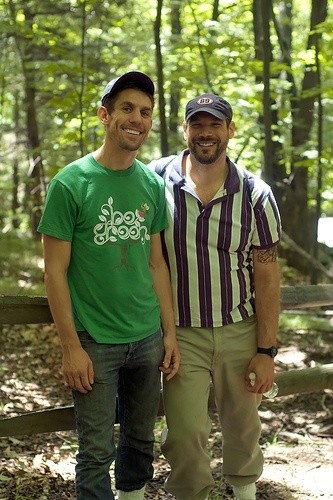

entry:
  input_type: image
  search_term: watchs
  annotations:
[256,346,278,358]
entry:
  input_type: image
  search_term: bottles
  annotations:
[249,373,279,399]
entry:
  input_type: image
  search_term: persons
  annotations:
[144,94,282,500]
[36,71,169,500]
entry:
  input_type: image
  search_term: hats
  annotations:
[100,71,156,102]
[183,94,234,122]
